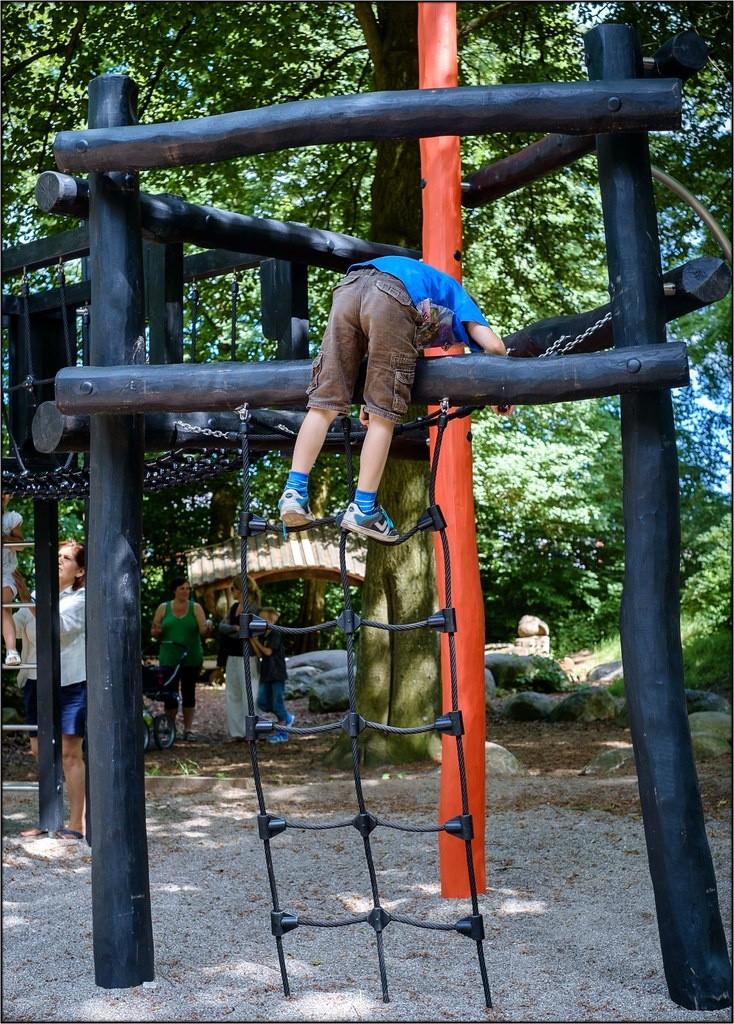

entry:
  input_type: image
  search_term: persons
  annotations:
[11,542,85,839]
[277,257,512,541]
[210,575,295,743]
[1,494,24,665]
[150,578,212,742]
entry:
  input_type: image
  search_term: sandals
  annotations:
[5,649,21,665]
[183,731,198,742]
[166,727,184,740]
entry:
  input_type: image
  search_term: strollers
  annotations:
[142,640,188,752]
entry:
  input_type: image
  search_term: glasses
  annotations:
[231,587,238,592]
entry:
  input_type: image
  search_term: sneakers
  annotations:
[278,489,314,532]
[266,732,289,744]
[341,503,399,541]
[286,713,294,727]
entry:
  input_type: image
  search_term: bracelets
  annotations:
[30,597,35,603]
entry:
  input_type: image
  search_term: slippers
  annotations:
[20,828,83,839]
